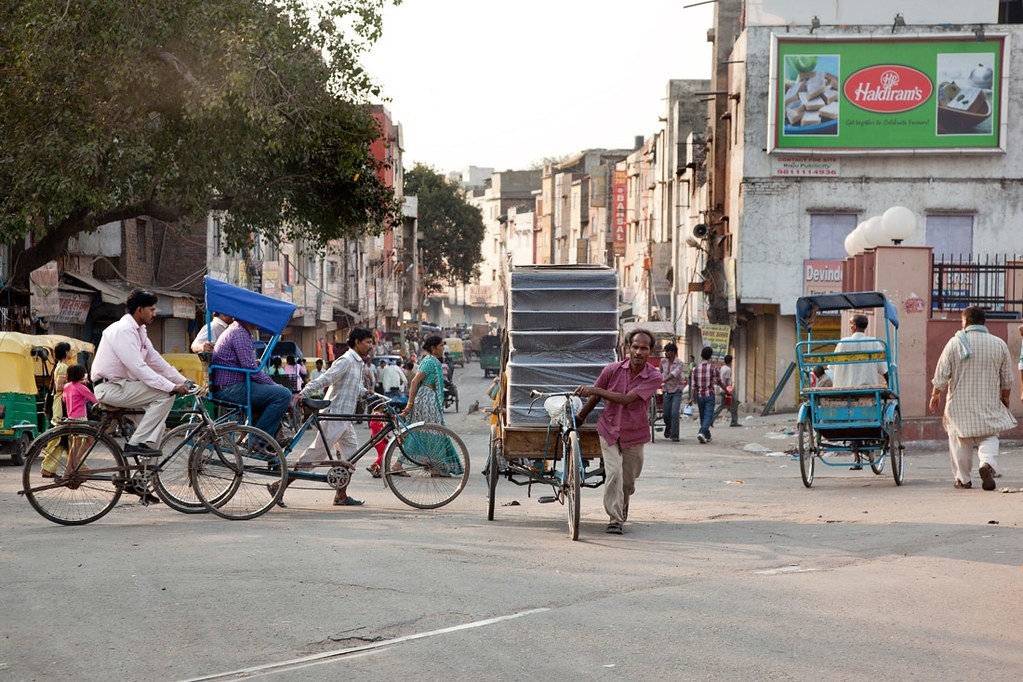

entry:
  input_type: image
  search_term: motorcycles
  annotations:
[160,353,216,424]
[0,330,98,467]
[441,337,465,368]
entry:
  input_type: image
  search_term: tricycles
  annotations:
[255,340,326,433]
[481,265,623,543]
[615,321,681,444]
[442,378,458,413]
[796,292,907,488]
[480,335,502,378]
[153,275,472,510]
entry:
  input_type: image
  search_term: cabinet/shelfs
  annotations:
[507,269,618,426]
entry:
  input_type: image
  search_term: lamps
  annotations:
[809,15,821,33]
[891,13,907,33]
[845,206,915,257]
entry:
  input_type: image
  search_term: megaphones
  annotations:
[693,223,708,238]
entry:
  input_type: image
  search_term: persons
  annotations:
[813,365,831,386]
[930,305,1014,491]
[572,331,663,534]
[40,288,505,506]
[1018,339,1023,403]
[660,343,742,445]
[832,316,889,470]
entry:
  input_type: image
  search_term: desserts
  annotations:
[938,81,986,113]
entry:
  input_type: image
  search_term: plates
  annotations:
[782,117,837,133]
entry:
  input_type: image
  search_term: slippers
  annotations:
[333,496,366,505]
[390,471,411,478]
[266,482,288,509]
[431,472,452,478]
[42,473,62,478]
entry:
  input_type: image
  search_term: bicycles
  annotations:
[22,382,289,526]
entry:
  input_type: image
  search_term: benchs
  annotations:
[797,338,892,425]
[185,352,282,464]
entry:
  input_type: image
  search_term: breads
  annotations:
[784,68,839,126]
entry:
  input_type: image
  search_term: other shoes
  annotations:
[979,463,996,490]
[664,430,670,438]
[366,464,379,478]
[954,480,972,489]
[243,438,277,458]
[606,523,624,535]
[623,504,629,523]
[122,443,163,458]
[705,433,712,442]
[697,431,708,444]
[672,437,680,442]
[124,479,160,503]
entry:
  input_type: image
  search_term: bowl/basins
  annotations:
[937,97,991,131]
